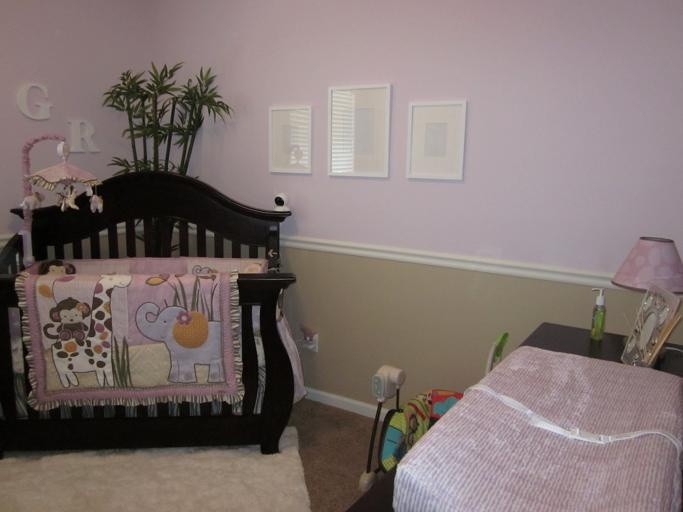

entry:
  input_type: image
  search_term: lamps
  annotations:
[611,236,683,294]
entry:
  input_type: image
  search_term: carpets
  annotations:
[1,427,312,512]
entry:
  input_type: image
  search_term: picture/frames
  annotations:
[267,84,466,181]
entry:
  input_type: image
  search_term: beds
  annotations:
[1,172,295,457]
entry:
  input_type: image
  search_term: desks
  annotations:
[343,322,682,512]
